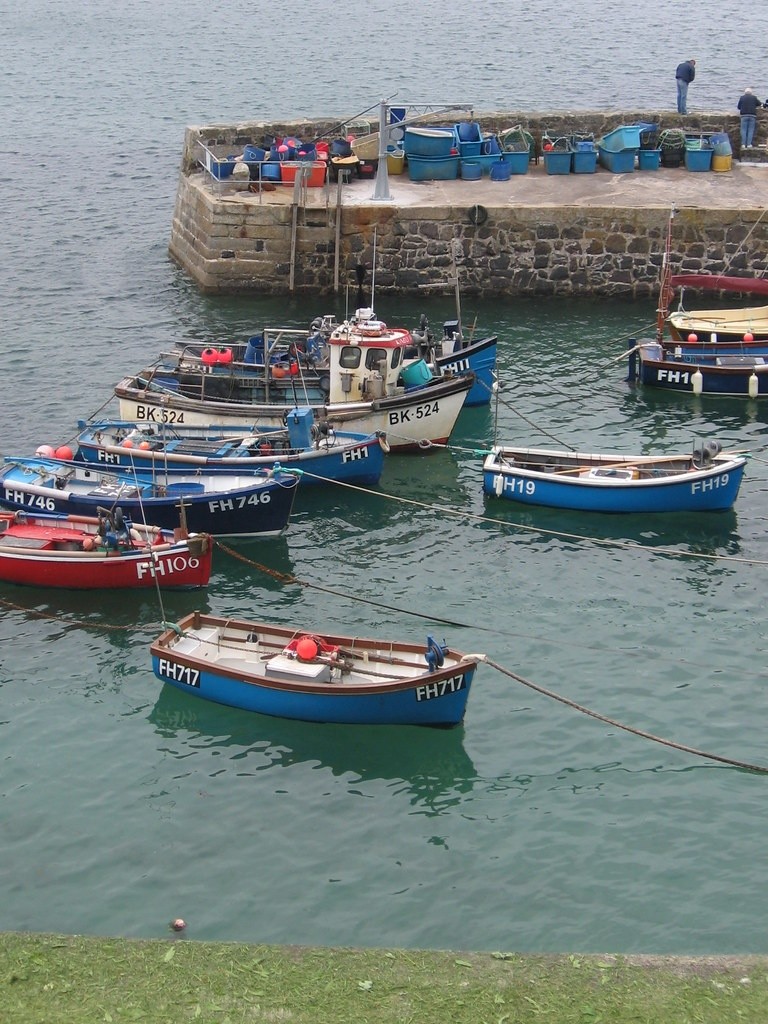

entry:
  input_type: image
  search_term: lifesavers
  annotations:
[356,320,387,332]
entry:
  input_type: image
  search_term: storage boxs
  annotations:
[211,120,734,193]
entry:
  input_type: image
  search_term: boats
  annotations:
[482,434,748,513]
[159,225,498,406]
[1,492,214,589]
[117,317,475,450]
[149,610,479,726]
[76,416,391,488]
[654,201,768,337]
[0,448,300,538]
[637,336,768,400]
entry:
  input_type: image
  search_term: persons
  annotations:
[419,313,429,331]
[737,88,763,149]
[675,59,696,115]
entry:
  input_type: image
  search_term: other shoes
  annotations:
[681,111,692,115]
[741,144,746,149]
[747,144,753,148]
